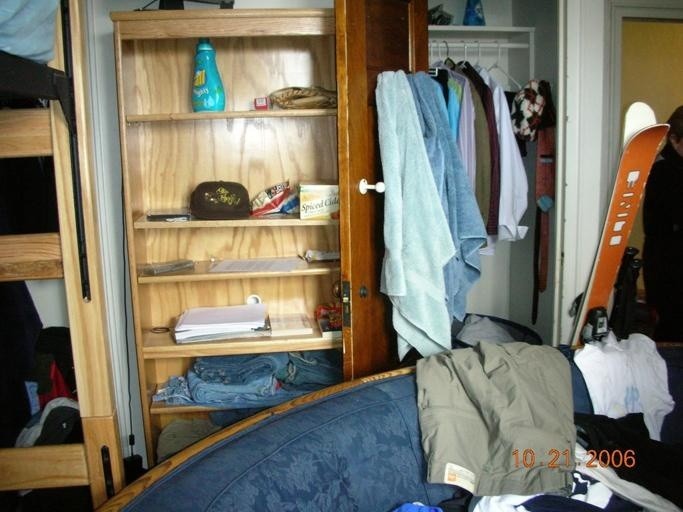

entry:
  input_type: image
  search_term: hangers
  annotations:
[423,39,524,96]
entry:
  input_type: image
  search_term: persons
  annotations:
[642,103,683,348]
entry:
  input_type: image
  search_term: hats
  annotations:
[511,80,545,143]
[189,181,249,220]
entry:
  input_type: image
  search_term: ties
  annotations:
[535,127,555,292]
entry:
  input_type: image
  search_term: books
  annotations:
[172,305,343,345]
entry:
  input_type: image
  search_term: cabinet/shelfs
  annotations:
[107,8,350,468]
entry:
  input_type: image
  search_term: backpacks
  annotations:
[14,397,84,497]
[33,327,78,411]
